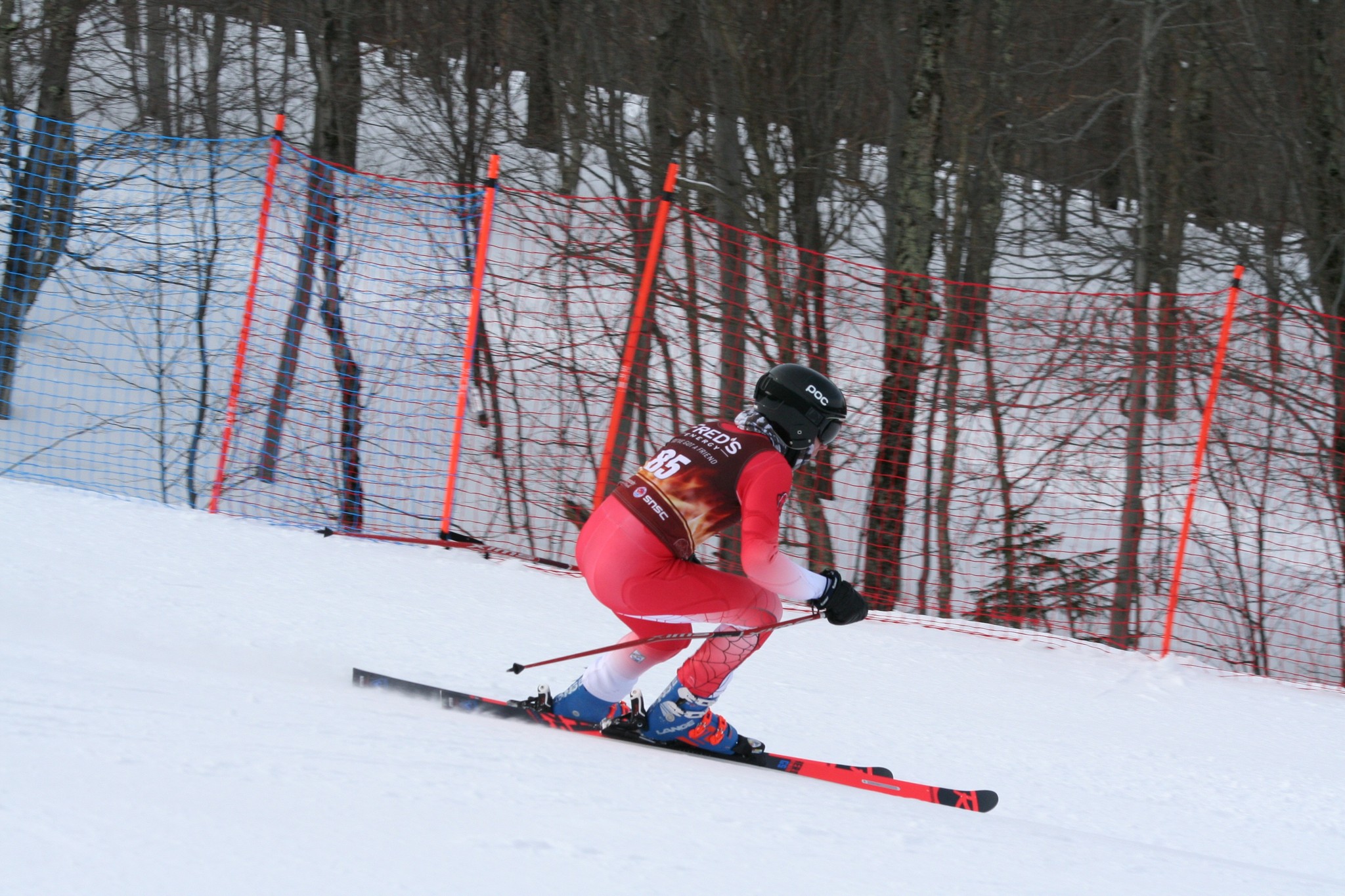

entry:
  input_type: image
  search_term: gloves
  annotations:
[807,568,869,625]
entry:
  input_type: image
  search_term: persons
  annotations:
[551,363,869,754]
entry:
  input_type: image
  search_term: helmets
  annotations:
[754,363,847,450]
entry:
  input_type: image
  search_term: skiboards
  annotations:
[350,665,1000,815]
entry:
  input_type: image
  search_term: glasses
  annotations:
[818,417,844,446]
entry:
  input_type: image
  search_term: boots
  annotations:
[639,674,738,755]
[549,674,633,724]
